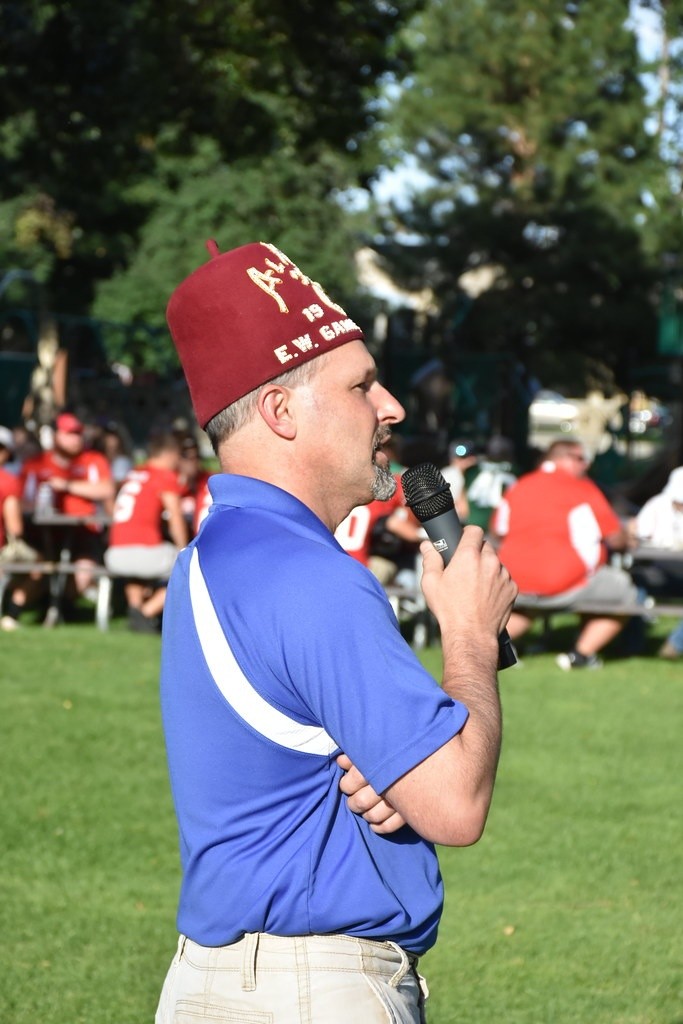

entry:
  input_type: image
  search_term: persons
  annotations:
[153,236,517,1023]
[1,414,683,672]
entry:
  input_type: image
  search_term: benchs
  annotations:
[382,588,683,663]
[0,561,169,632]
[478,535,681,566]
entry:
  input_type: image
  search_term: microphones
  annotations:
[399,462,517,669]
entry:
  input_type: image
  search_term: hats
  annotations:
[165,238,366,429]
